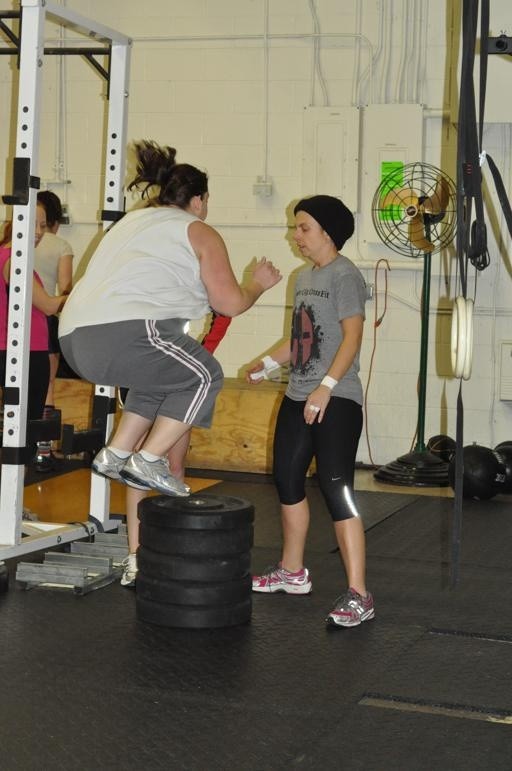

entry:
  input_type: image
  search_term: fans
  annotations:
[373,162,464,490]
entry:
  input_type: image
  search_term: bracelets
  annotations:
[321,376,338,391]
[260,355,273,369]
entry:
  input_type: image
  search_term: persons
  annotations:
[0,199,71,449]
[34,191,74,460]
[245,195,376,628]
[57,137,283,497]
[120,194,232,586]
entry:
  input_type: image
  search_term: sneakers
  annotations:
[120,553,138,586]
[36,455,55,472]
[92,448,190,497]
[251,561,313,594]
[325,588,375,628]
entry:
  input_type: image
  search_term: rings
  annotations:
[314,407,320,413]
[309,405,314,410]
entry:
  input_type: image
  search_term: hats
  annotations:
[295,195,354,251]
